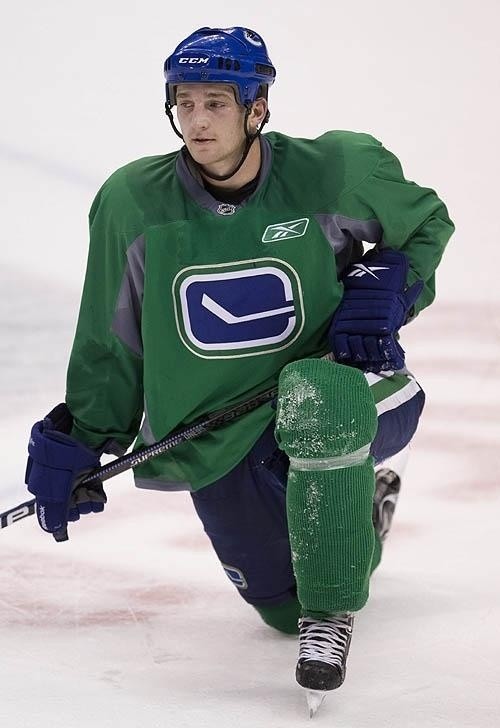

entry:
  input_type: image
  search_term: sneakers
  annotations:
[369,465,403,545]
[292,601,354,694]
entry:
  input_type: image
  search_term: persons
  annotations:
[24,20,459,693]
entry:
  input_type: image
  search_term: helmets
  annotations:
[160,22,276,110]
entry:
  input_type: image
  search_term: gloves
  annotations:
[22,398,112,543]
[327,246,424,376]
